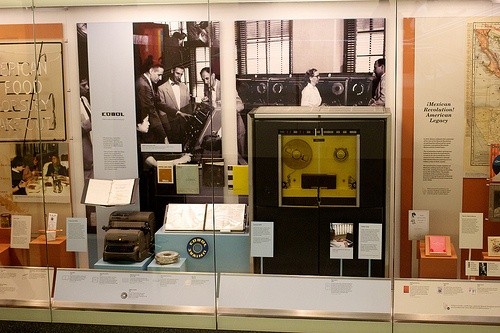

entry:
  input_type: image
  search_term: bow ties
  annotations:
[209,88,215,92]
[171,82,179,86]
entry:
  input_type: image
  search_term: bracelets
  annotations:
[18,184,20,189]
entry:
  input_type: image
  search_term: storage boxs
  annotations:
[94,251,155,269]
[146,224,252,271]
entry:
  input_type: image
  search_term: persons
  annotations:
[135,61,246,230]
[300,68,329,106]
[330,229,348,248]
[79,73,97,233]
[11,154,41,195]
[47,154,68,176]
[368,59,386,106]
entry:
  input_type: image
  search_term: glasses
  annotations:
[313,75,319,78]
